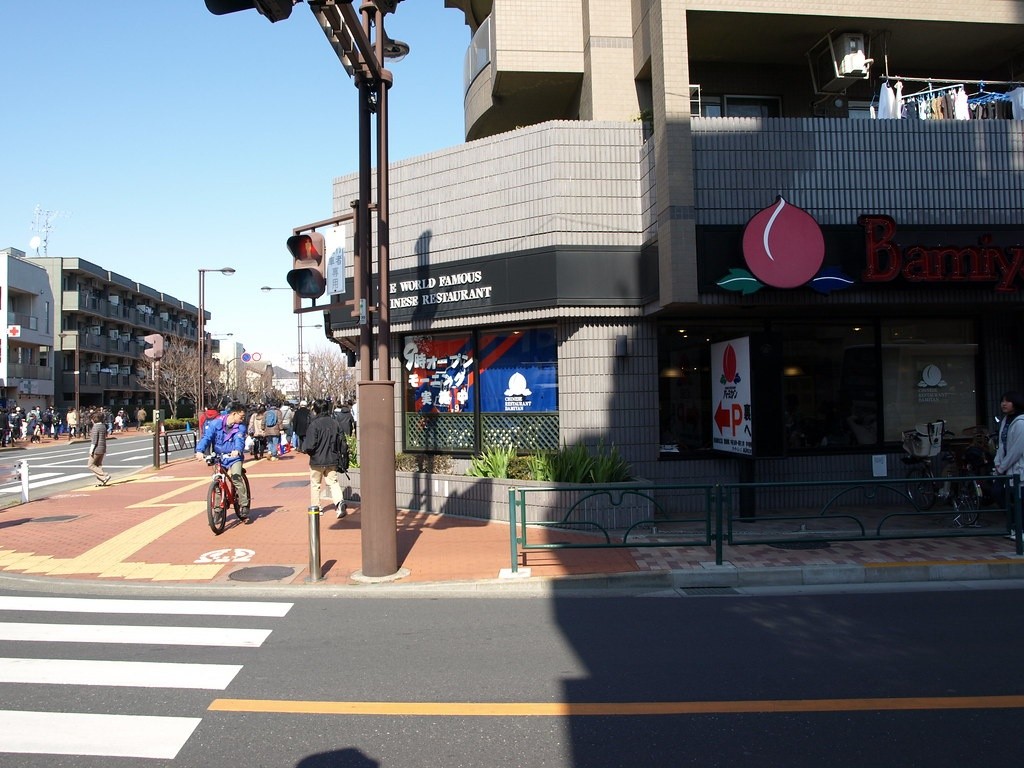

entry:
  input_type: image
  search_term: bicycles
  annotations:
[902,428,987,528]
[199,453,254,535]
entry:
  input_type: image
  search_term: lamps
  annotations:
[783,353,805,377]
[660,342,686,377]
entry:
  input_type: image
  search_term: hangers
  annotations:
[966,82,990,97]
[870,91,880,109]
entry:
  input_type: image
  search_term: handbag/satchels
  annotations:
[277,443,281,456]
[245,435,254,451]
[280,445,285,454]
[337,458,348,473]
[281,434,291,453]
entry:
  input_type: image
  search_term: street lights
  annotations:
[58,332,81,438]
[261,285,323,408]
[342,0,411,581]
[196,266,236,458]
[207,331,234,410]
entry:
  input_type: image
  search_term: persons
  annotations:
[195,405,250,524]
[87,412,112,487]
[198,396,358,463]
[301,398,349,519]
[993,391,1024,543]
[0,405,147,448]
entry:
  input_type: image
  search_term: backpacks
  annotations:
[266,410,276,427]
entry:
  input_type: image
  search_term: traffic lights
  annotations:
[142,333,164,359]
[287,231,327,306]
[204,0,292,23]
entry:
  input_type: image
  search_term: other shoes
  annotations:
[319,509,322,515]
[96,483,103,487]
[241,507,250,517]
[337,501,347,518]
[214,517,222,525]
[103,475,111,484]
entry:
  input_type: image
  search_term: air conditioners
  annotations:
[815,33,867,94]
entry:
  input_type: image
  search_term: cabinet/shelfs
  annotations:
[689,84,701,116]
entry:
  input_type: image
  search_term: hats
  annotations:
[300,401,307,405]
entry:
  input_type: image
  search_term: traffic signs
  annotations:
[708,337,753,457]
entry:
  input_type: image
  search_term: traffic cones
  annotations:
[159,423,166,436]
